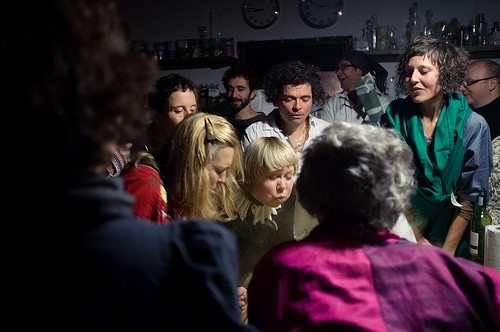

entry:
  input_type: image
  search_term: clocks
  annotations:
[299,0,344,29]
[241,0,280,29]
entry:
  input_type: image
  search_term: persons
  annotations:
[0,0,500,332]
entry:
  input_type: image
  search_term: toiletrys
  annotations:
[362,14,396,52]
[438,13,500,47]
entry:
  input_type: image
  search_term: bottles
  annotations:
[470,188,492,266]
[151,48,159,70]
[363,14,380,51]
[408,3,433,45]
[214,32,224,56]
[182,41,189,58]
[446,12,500,47]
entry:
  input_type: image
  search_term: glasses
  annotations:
[334,64,352,74]
[467,76,498,86]
